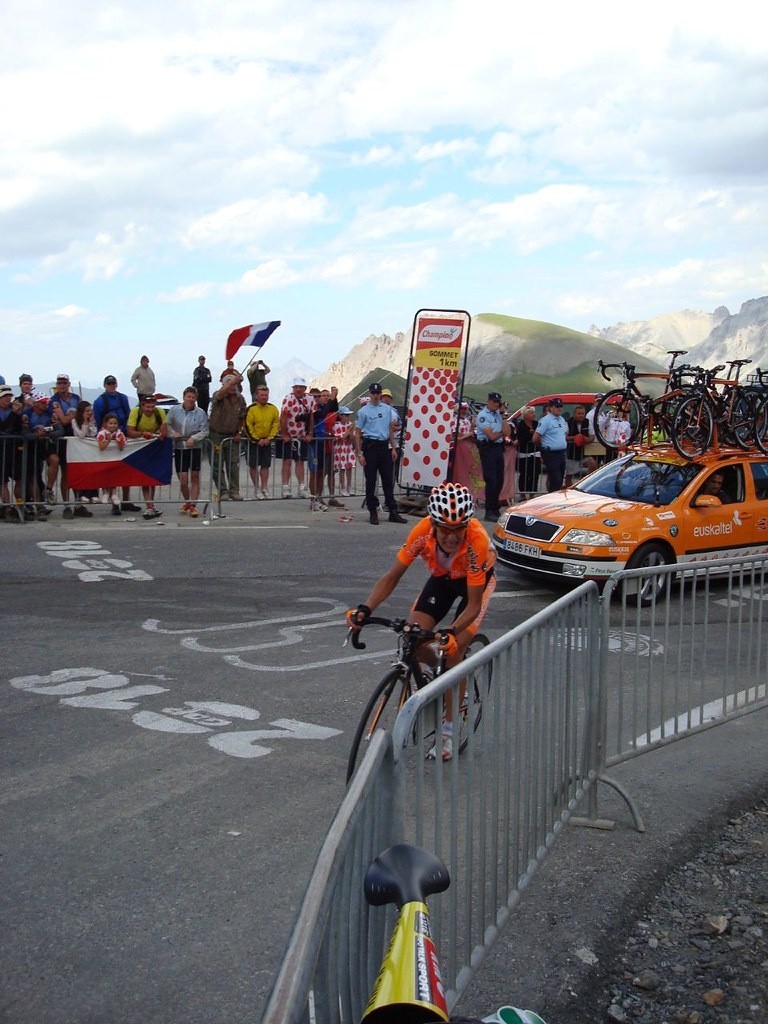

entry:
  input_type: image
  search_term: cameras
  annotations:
[299,413,307,422]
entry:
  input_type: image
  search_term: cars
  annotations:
[491,439,768,609]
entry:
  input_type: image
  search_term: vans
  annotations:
[506,392,661,441]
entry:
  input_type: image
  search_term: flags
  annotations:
[225,320,281,361]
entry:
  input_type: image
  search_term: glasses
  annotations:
[370,392,381,394]
[550,405,563,408]
[106,381,116,385]
[434,523,467,535]
[145,396,156,400]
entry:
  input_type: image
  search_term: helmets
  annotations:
[427,483,475,529]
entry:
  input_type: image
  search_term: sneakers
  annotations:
[429,731,454,760]
[423,643,440,678]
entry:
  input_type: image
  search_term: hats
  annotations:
[292,377,306,387]
[104,376,116,383]
[0,384,14,398]
[489,392,502,401]
[594,394,603,401]
[549,398,563,406]
[369,382,382,392]
[339,407,354,414]
[381,388,393,398]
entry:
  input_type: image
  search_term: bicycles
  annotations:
[462,393,510,427]
[342,605,493,788]
[355,844,549,1024]
[592,349,768,460]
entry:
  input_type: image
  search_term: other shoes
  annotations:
[518,496,527,502]
[389,510,408,524]
[370,512,379,525]
[484,508,503,523]
[0,487,355,523]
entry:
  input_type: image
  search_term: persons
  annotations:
[532,397,569,493]
[604,409,631,463]
[168,386,208,515]
[243,385,280,500]
[131,356,156,401]
[248,359,271,403]
[333,406,355,497]
[0,373,167,523]
[586,394,606,464]
[517,406,541,501]
[346,482,498,760]
[452,401,475,434]
[355,383,402,525]
[208,375,246,501]
[475,391,511,522]
[276,378,314,499]
[193,356,212,415]
[643,409,668,443]
[565,406,597,488]
[309,386,344,511]
[220,361,244,392]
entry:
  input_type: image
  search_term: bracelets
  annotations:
[502,418,506,420]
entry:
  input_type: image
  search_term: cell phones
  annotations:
[43,427,54,432]
